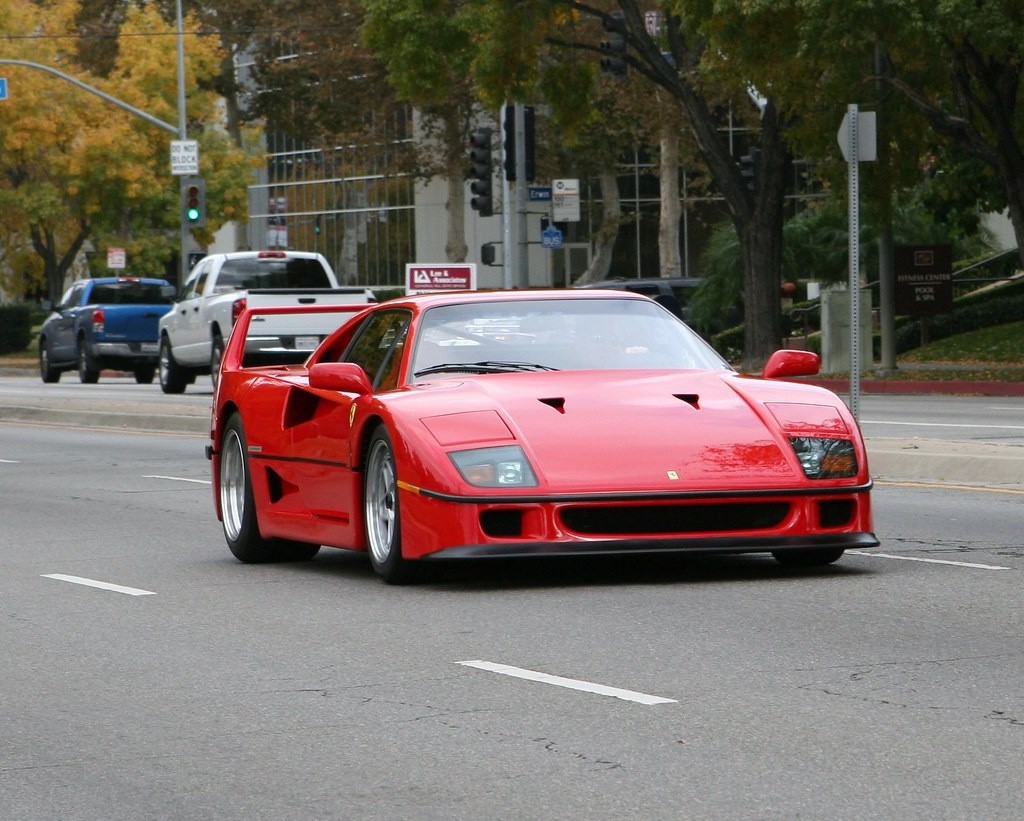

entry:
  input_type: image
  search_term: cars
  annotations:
[575,275,741,359]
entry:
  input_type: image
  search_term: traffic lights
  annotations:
[504,106,536,181]
[181,178,206,228]
[465,128,493,218]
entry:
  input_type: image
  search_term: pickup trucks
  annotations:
[37,277,178,384]
[157,249,377,392]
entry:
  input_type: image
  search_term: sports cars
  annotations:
[204,289,885,588]
